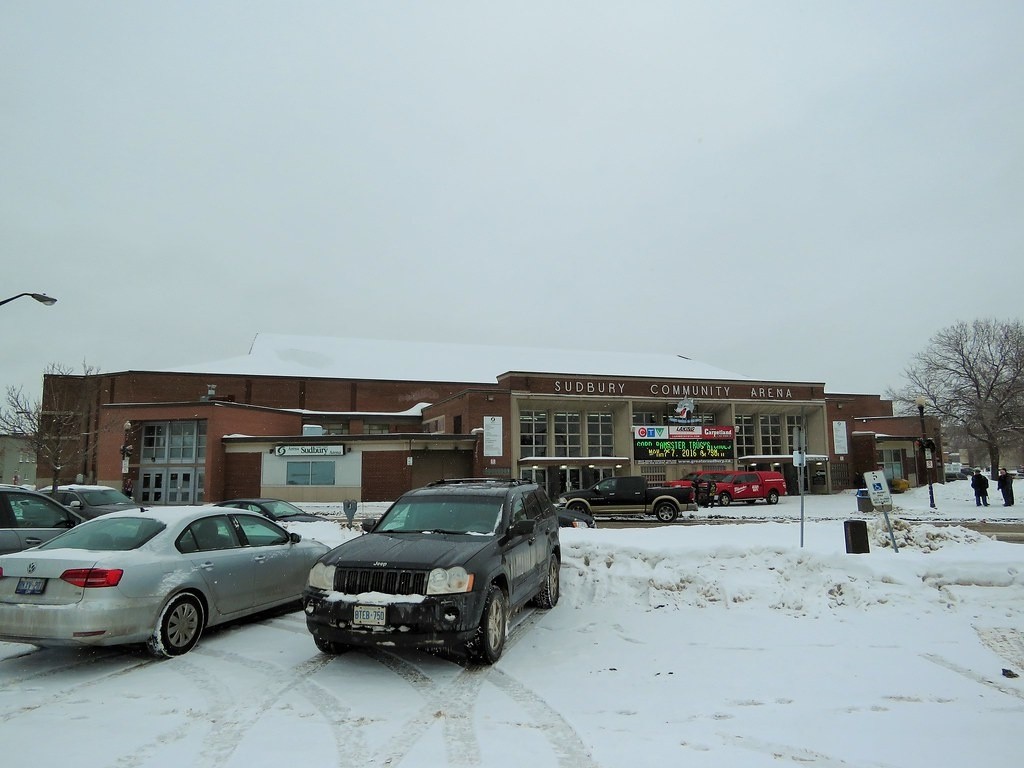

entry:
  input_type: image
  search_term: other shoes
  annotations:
[1003,503,1011,506]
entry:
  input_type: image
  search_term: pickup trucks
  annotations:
[704,470,788,507]
[663,470,729,504]
[558,475,699,524]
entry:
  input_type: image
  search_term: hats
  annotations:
[971,468,980,473]
[709,475,713,479]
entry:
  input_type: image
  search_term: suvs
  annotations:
[301,476,562,665]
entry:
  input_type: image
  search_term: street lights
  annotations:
[122,421,133,497]
[917,396,938,510]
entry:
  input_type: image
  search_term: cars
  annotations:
[0,506,324,660]
[37,483,142,524]
[208,497,365,550]
[946,467,980,481]
[1,483,88,555]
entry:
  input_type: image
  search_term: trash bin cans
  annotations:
[856,488,874,513]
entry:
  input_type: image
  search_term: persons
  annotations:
[703,479,718,507]
[691,474,703,507]
[12,470,21,485]
[997,468,1014,506]
[971,469,990,506]
[123,475,134,499]
